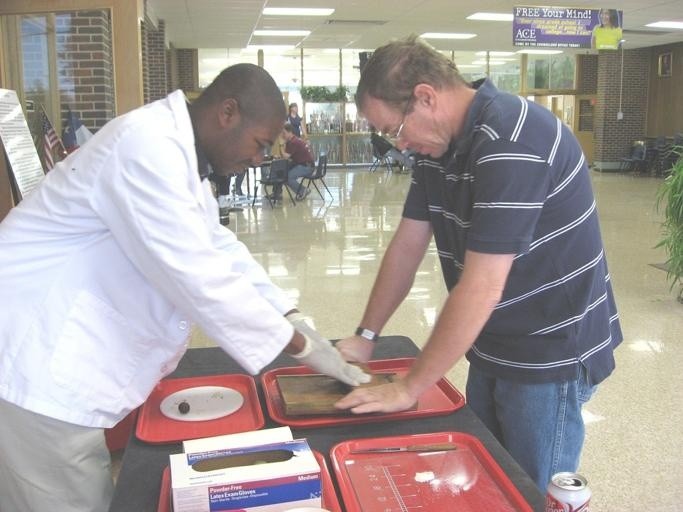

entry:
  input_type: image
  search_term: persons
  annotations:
[219,175,245,195]
[334,32,624,496]
[380,130,414,168]
[591,9,623,50]
[265,103,316,200]
[0,64,371,512]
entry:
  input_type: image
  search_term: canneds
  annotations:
[545,472,592,512]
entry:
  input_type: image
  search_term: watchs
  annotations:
[355,328,381,343]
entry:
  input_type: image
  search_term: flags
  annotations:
[41,114,60,174]
[61,113,94,148]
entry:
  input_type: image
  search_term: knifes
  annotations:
[351,444,458,456]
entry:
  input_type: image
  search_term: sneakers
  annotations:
[297,188,311,200]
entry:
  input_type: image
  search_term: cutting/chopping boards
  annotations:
[273,372,417,417]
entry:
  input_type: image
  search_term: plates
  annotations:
[159,385,244,420]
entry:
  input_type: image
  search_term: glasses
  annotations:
[375,92,414,148]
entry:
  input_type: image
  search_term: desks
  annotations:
[103,328,549,511]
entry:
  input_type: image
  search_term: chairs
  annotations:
[242,141,408,209]
[617,133,683,182]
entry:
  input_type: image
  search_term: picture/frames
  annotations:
[657,52,672,78]
